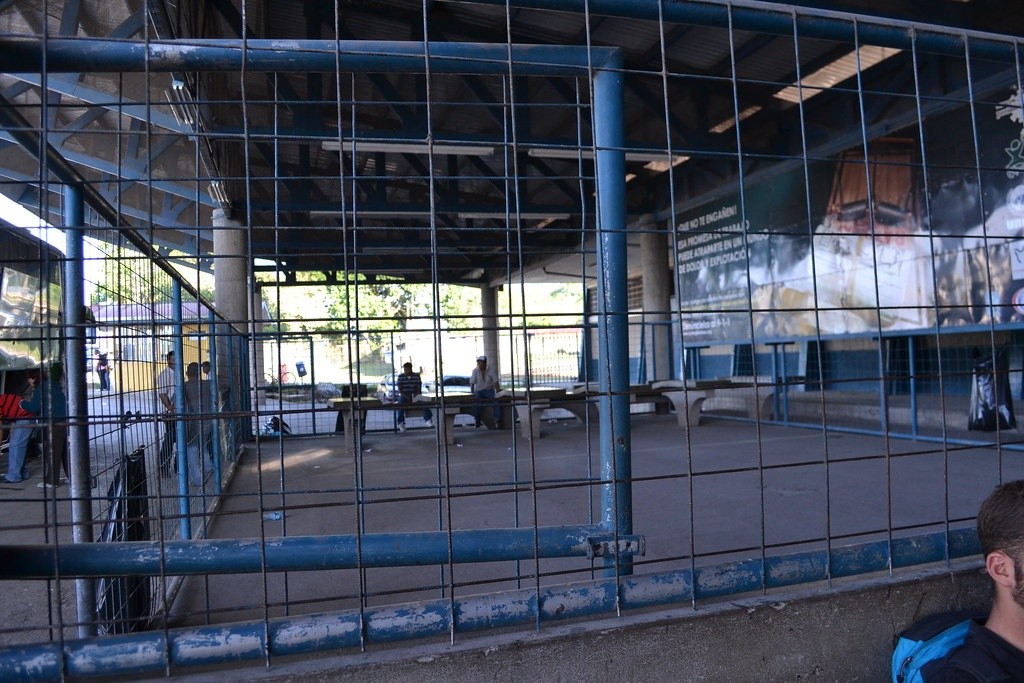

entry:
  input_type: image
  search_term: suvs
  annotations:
[378,372,401,402]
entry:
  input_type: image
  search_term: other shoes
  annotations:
[96,388,106,392]
[0,473,22,483]
[64,479,70,484]
[425,420,433,427]
[475,421,483,428]
[157,471,171,479]
[495,421,500,429]
[37,482,58,488]
[398,423,406,432]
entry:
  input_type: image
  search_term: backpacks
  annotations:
[891,609,989,683]
[99,354,107,366]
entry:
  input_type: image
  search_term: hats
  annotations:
[477,356,487,362]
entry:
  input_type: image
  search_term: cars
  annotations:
[429,375,474,393]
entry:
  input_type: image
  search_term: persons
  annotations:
[0,394,35,483]
[158,351,174,477]
[397,362,433,432]
[930,480,1024,683]
[94,347,113,392]
[171,362,213,473]
[470,356,504,429]
[18,369,68,487]
[202,362,211,380]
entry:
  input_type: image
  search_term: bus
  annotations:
[1,191,97,459]
